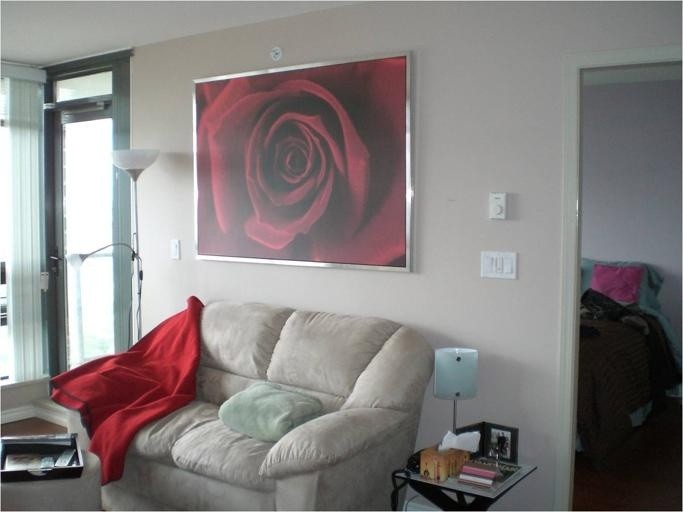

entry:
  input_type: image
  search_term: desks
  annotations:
[393,459,540,510]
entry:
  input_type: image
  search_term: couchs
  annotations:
[65,300,435,511]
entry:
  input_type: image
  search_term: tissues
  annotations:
[418,430,482,484]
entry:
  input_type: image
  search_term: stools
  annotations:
[1,449,101,512]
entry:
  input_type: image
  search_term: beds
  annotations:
[576,260,670,473]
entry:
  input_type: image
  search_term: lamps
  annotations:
[434,347,478,434]
[62,148,160,342]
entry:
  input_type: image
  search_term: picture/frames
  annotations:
[190,52,411,273]
[455,422,519,464]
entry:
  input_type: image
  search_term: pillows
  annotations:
[218,381,322,443]
[593,263,644,305]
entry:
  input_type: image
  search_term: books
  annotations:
[457,463,497,489]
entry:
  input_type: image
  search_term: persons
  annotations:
[488,431,510,459]
